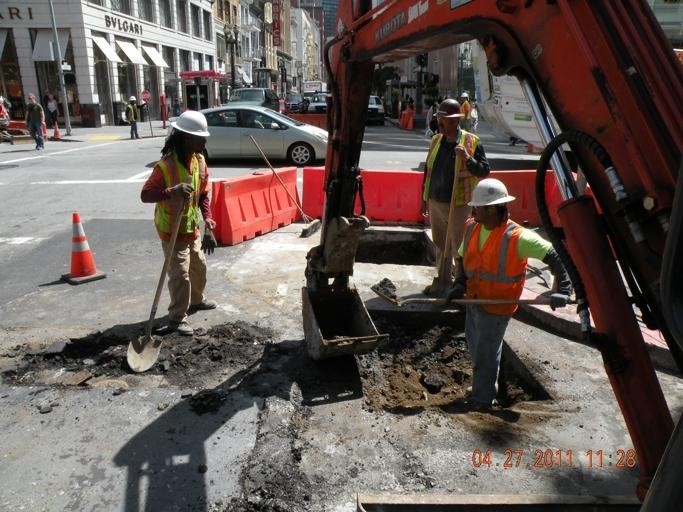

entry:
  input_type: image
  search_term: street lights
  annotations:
[277,59,287,97]
[223,23,238,89]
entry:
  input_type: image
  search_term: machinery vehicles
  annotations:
[302,0,681,510]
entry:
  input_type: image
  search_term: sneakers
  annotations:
[188,298,217,311]
[9,136,14,145]
[454,397,491,411]
[167,318,194,335]
[466,385,473,395]
[37,146,44,150]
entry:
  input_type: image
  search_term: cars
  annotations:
[164,104,329,166]
[284,81,328,114]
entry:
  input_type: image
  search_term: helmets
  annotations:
[128,96,136,102]
[432,99,465,119]
[466,178,517,207]
[170,110,211,138]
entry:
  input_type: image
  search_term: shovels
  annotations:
[125,192,189,373]
[370,275,581,308]
[429,149,464,296]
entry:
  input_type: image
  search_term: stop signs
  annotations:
[141,91,150,101]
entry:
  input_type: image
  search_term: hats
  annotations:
[460,92,469,98]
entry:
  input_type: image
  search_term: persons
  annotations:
[26,92,47,150]
[138,109,219,337]
[47,94,61,128]
[419,98,492,296]
[426,99,441,137]
[471,101,479,132]
[173,99,181,117]
[0,95,16,145]
[443,175,574,410]
[126,95,149,139]
[459,92,472,132]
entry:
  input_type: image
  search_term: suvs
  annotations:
[365,95,384,125]
[225,88,279,112]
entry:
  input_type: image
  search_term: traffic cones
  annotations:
[61,211,106,286]
[405,112,414,130]
[50,121,61,140]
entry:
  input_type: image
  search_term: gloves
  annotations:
[549,294,570,310]
[444,283,466,311]
[200,227,218,255]
[173,183,195,198]
